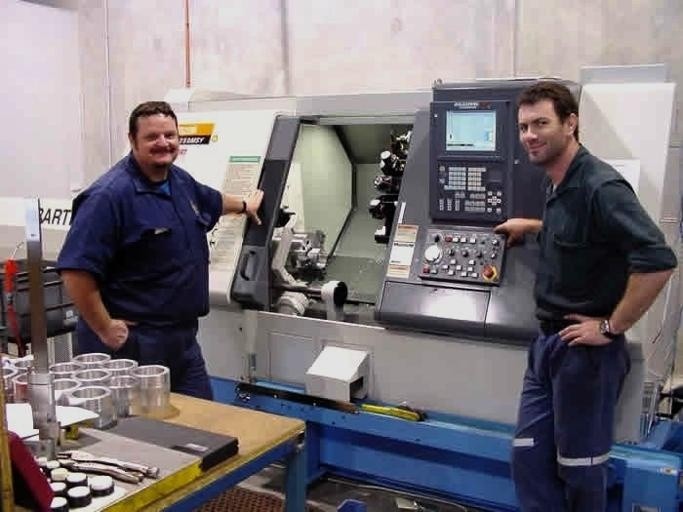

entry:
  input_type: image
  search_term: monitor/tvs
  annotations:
[445,108,496,153]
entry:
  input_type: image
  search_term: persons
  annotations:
[490,81,678,511]
[52,101,267,403]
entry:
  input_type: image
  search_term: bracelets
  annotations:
[236,199,248,216]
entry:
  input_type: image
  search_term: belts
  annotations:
[539,317,581,334]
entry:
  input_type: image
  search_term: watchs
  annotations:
[598,315,623,343]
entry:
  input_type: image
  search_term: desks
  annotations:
[1,389,309,512]
